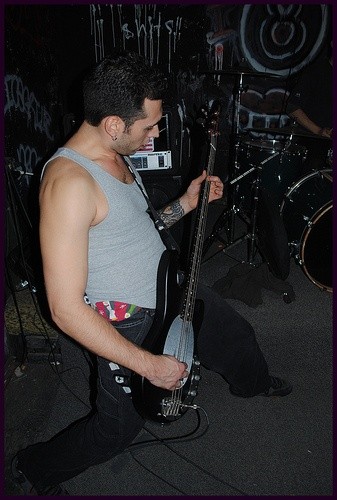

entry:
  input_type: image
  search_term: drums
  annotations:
[236,136,309,169]
[277,169,334,294]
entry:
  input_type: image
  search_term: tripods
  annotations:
[201,76,292,304]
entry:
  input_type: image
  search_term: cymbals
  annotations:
[204,68,283,79]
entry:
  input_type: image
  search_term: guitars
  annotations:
[132,100,225,425]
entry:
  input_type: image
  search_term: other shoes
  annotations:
[8,454,71,497]
[258,375,292,397]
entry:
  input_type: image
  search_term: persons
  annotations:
[194,4,257,203]
[285,38,334,258]
[0,51,293,500]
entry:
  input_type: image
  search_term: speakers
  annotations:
[140,172,193,289]
